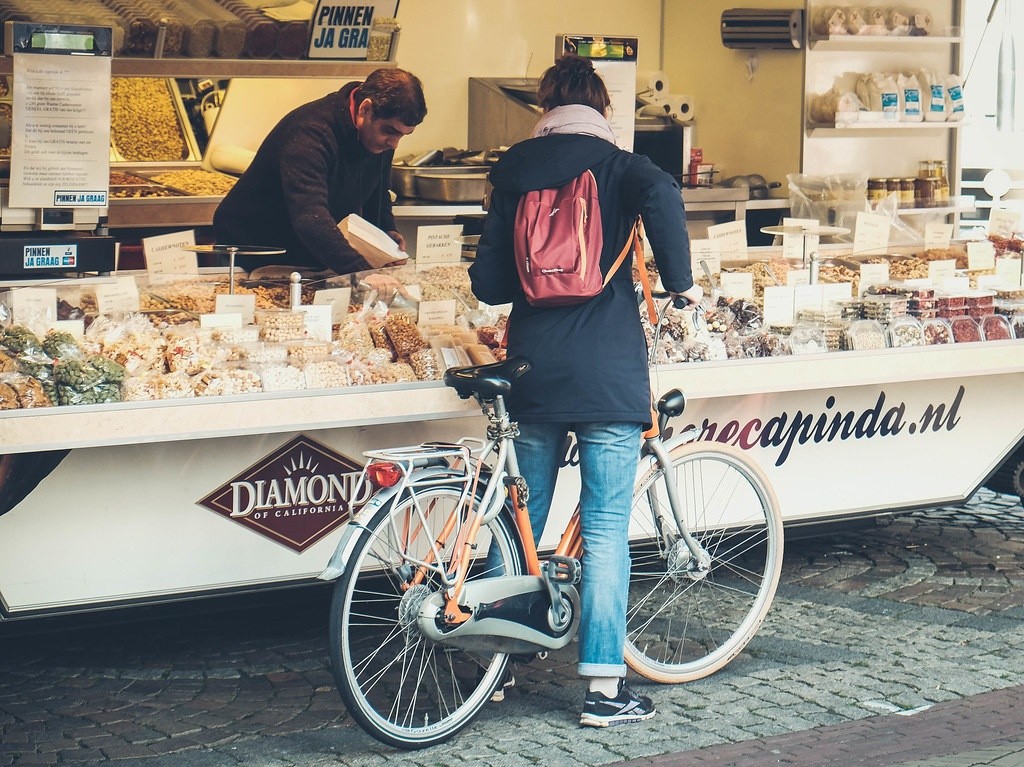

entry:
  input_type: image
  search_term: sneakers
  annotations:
[475,664,516,702]
[580,677,657,728]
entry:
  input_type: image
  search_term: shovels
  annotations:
[358,278,430,321]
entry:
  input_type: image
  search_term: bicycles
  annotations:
[315,282,786,749]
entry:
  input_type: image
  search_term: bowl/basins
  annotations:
[392,166,492,197]
[414,174,485,202]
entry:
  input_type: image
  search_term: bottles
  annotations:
[918,160,950,208]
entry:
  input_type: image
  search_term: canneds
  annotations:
[869,160,950,209]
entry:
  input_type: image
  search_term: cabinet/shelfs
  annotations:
[0,0,400,226]
[806,0,966,138]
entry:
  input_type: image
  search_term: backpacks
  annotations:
[513,169,640,306]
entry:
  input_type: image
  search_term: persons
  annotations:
[212,67,428,271]
[468,53,705,727]
